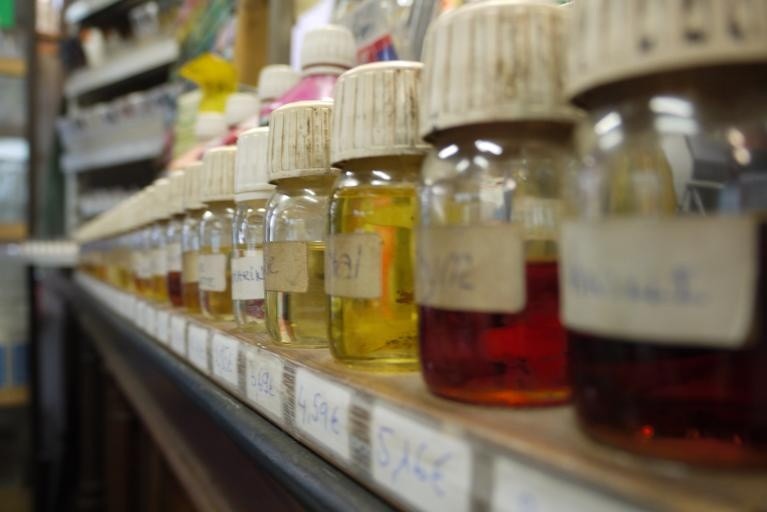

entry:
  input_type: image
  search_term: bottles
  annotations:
[65,0,766,492]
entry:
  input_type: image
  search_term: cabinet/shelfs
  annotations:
[1,0,296,512]
